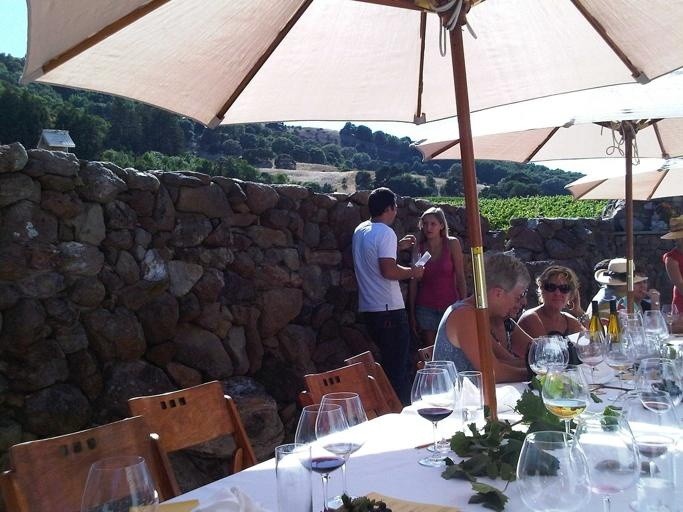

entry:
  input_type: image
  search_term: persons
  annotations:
[661,215,683,333]
[432,255,660,394]
[351,186,425,406]
[409,207,467,345]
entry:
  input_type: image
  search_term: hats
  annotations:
[594,258,648,286]
[660,215,683,240]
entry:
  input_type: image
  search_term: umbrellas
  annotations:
[409,74,683,312]
[564,158,683,202]
[18,0,683,417]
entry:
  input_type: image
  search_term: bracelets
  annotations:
[409,267,414,279]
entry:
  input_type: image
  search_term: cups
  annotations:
[458,370,485,436]
[639,290,659,304]
[275,442,312,512]
[80,456,159,512]
[516,431,590,510]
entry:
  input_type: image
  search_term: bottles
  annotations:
[607,300,621,342]
[589,301,606,341]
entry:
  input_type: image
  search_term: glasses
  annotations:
[541,283,572,294]
[496,285,529,301]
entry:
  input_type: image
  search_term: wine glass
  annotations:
[618,304,679,359]
[411,359,461,468]
[576,331,608,396]
[602,333,637,401]
[529,334,570,374]
[571,415,641,512]
[295,390,369,511]
[620,359,681,512]
[541,365,590,431]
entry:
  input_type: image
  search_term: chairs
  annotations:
[0,380,258,512]
[295,350,404,431]
